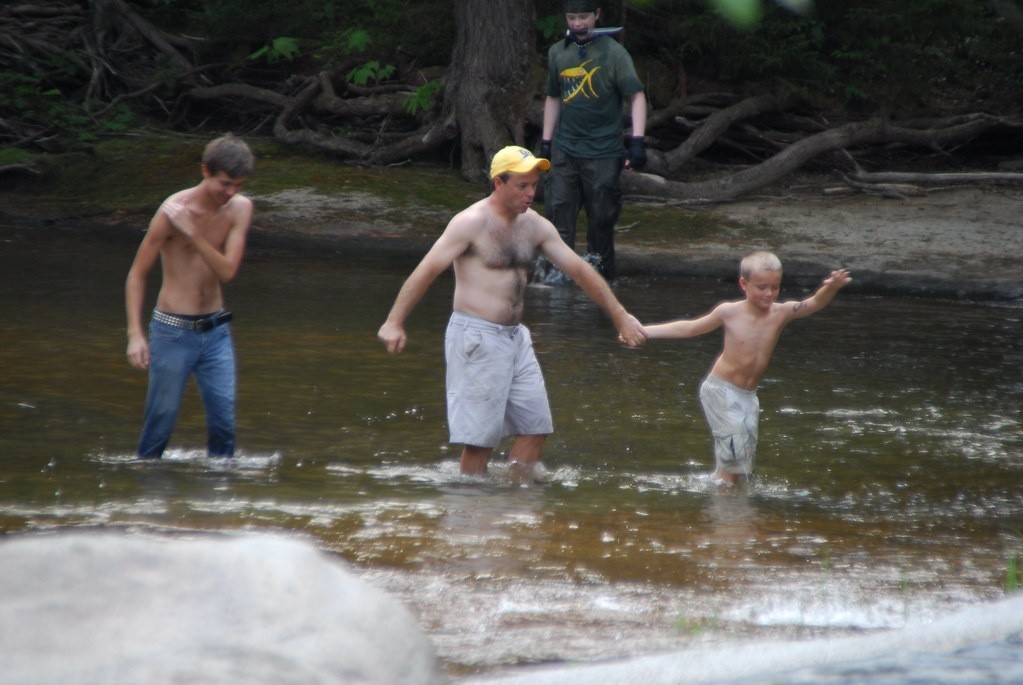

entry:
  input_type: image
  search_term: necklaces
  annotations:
[573,40,592,47]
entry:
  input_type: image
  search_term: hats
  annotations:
[490,145,550,180]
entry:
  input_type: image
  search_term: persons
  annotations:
[619,251,852,486]
[537,0,647,278]
[125,132,255,457]
[377,146,648,475]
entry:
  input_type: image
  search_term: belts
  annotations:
[153,309,233,333]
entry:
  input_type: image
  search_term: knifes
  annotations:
[570,27,623,33]
